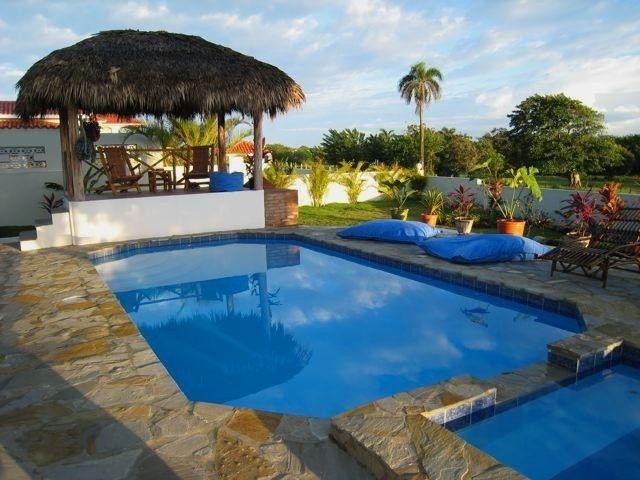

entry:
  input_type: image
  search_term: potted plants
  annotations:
[378,178,475,235]
[468,157,543,238]
[555,187,609,248]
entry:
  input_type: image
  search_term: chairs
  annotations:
[536,208,640,287]
[183,144,214,191]
[97,144,144,196]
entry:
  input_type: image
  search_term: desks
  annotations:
[148,170,172,193]
